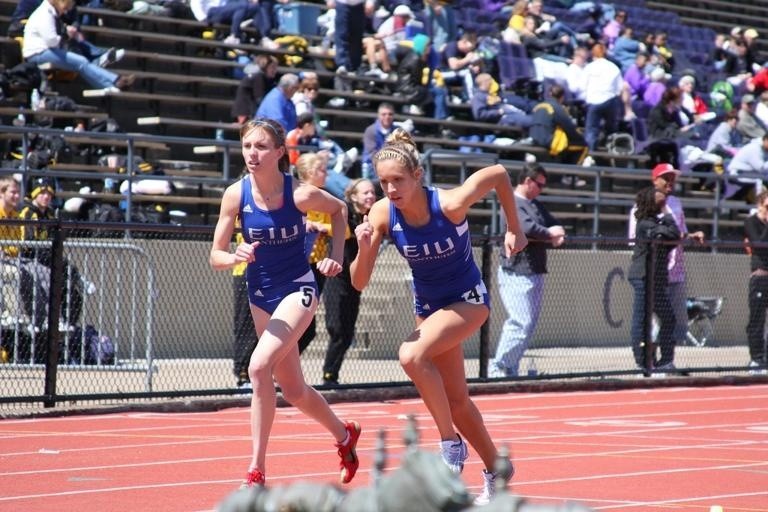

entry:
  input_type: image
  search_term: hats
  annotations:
[393,5,417,18]
[744,29,759,38]
[741,94,756,104]
[731,27,743,36]
[652,163,681,179]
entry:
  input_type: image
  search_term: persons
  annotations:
[349,127,529,508]
[208,115,362,492]
[628,187,688,377]
[0,175,75,344]
[18,183,85,344]
[271,152,352,357]
[742,188,768,376]
[1,1,768,254]
[322,177,376,386]
[490,161,566,378]
[231,166,262,389]
[626,163,705,375]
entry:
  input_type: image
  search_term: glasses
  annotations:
[532,178,546,188]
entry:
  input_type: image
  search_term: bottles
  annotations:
[216,120,225,140]
[31,88,40,111]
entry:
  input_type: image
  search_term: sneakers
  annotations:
[235,370,339,389]
[239,467,265,490]
[439,433,469,476]
[409,105,425,116]
[748,361,767,374]
[111,48,125,64]
[225,36,393,79]
[114,74,136,89]
[657,364,684,374]
[1,277,96,334]
[487,360,512,378]
[561,176,587,188]
[578,33,590,43]
[622,113,637,123]
[334,419,361,484]
[473,456,515,507]
